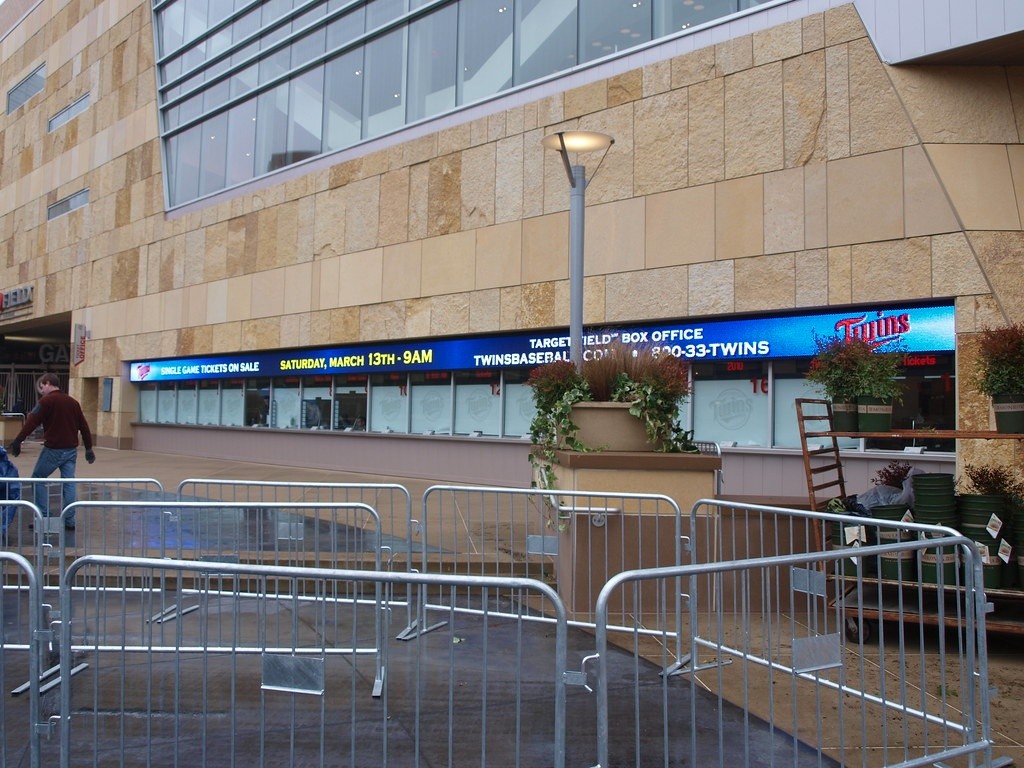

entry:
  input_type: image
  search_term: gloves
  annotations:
[9,439,20,457]
[85,449,95,464]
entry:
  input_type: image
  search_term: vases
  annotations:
[991,394,1024,433]
[857,394,894,432]
[830,394,857,432]
[553,399,662,452]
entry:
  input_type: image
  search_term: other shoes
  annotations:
[65,525,75,530]
[28,523,34,529]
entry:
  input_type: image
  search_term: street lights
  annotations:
[539,127,614,394]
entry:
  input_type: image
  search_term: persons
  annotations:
[12,398,28,418]
[7,374,95,532]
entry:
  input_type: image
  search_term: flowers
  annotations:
[969,325,1024,397]
[805,336,903,406]
[962,465,1024,510]
[871,460,912,491]
[525,348,697,547]
